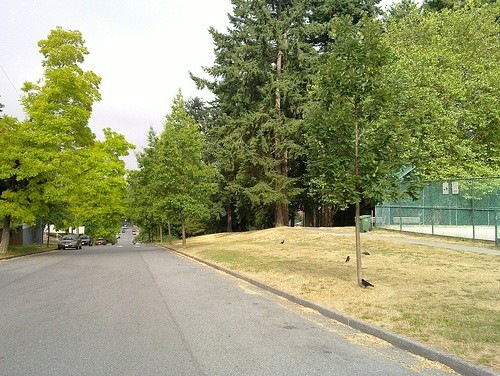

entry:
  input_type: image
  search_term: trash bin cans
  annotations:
[358,214,371,233]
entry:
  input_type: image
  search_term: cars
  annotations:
[58,234,81,250]
[95,238,107,245]
[80,235,92,246]
